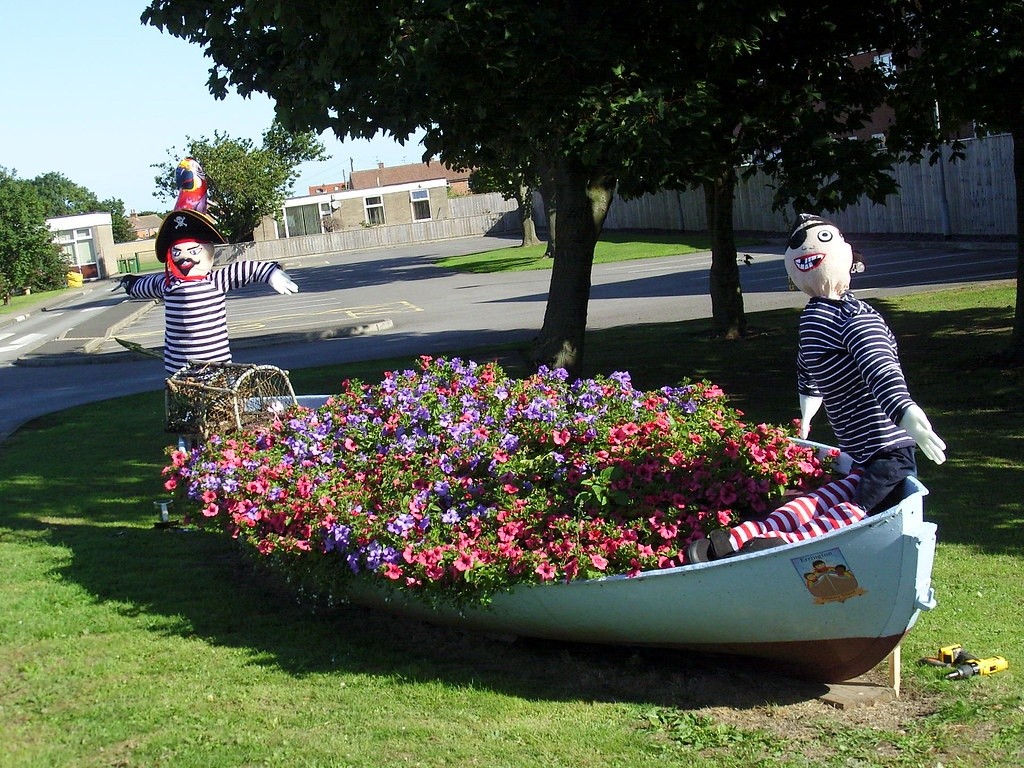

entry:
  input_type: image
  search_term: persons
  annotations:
[685,212,949,568]
[118,207,299,434]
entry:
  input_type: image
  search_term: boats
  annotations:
[214,394,941,688]
